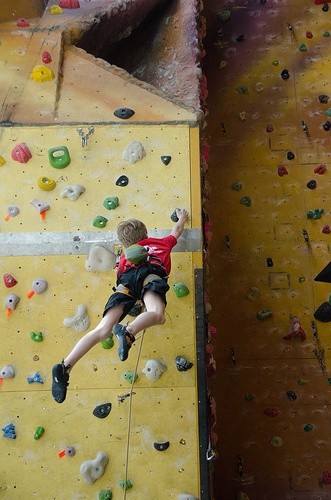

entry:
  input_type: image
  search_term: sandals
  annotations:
[51,358,72,403]
[112,321,136,362]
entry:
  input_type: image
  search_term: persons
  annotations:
[51,207,191,404]
